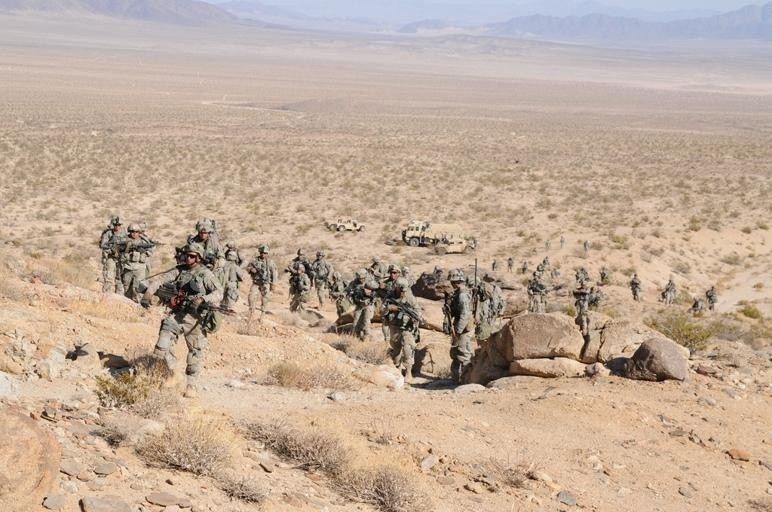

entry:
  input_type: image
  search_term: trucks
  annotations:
[402,220,476,255]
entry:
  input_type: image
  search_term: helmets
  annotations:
[107,217,146,232]
[195,218,215,233]
[257,244,270,254]
[226,240,238,261]
[356,255,401,278]
[297,248,307,255]
[316,250,324,257]
[183,243,206,261]
[446,269,498,288]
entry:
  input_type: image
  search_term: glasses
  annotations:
[187,254,198,259]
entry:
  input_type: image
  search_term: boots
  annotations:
[403,369,414,384]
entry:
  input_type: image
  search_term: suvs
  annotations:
[323,215,368,234]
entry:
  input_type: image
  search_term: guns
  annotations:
[110,240,167,252]
[109,216,121,259]
[136,281,239,318]
[471,257,479,315]
[247,261,271,287]
[387,295,434,333]
[290,273,302,296]
[444,289,458,347]
[348,286,380,297]
